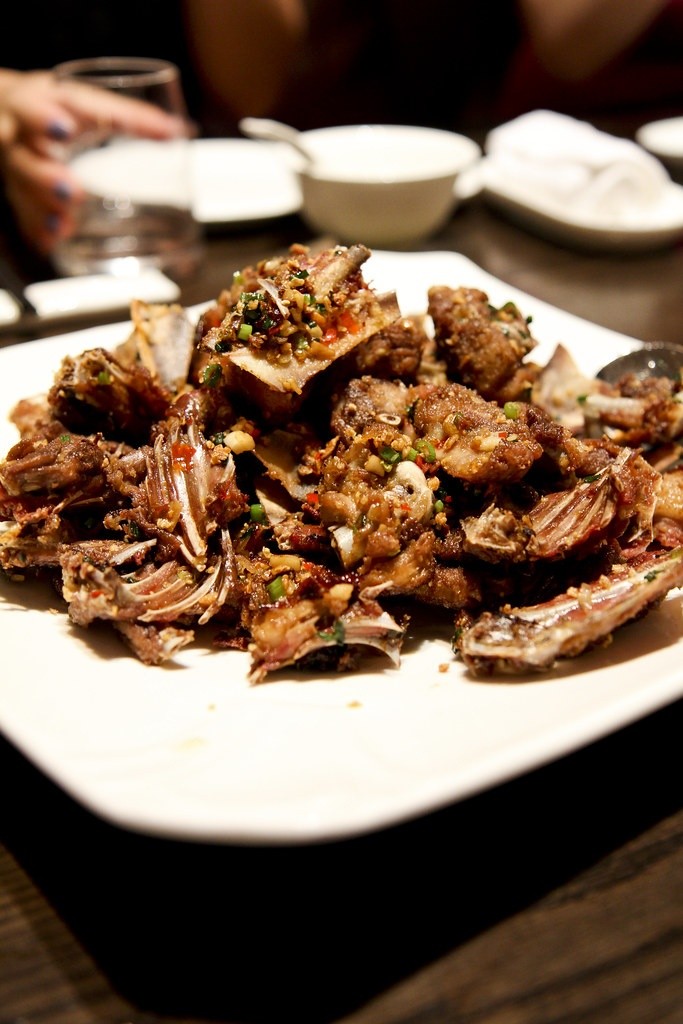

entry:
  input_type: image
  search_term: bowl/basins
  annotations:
[270,123,482,251]
[636,115,683,175]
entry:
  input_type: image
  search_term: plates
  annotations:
[70,135,303,226]
[0,248,683,846]
[476,140,683,255]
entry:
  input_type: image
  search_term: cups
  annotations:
[48,54,204,289]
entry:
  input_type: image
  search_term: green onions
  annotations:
[96,288,522,645]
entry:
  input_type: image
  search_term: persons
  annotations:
[0,0,683,253]
